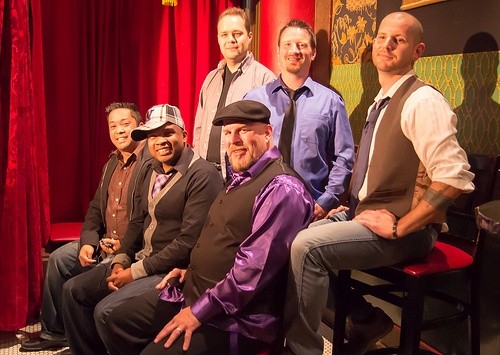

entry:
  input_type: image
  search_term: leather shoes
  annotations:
[53,349,73,355]
[21,336,69,350]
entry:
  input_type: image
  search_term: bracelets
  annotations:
[392,218,399,240]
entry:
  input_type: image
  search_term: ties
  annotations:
[346,97,390,221]
[278,86,307,167]
[152,171,172,199]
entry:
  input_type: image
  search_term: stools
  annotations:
[49,221,84,252]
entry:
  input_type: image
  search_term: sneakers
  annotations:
[343,306,394,355]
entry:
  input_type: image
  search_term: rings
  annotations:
[176,327,183,335]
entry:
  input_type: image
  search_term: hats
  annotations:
[212,100,270,126]
[130,104,184,142]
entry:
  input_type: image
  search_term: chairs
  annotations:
[332,148,500,355]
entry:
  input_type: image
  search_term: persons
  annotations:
[61,103,225,355]
[228,19,354,220]
[190,8,278,185]
[283,11,467,354]
[89,101,314,355]
[20,102,158,351]
